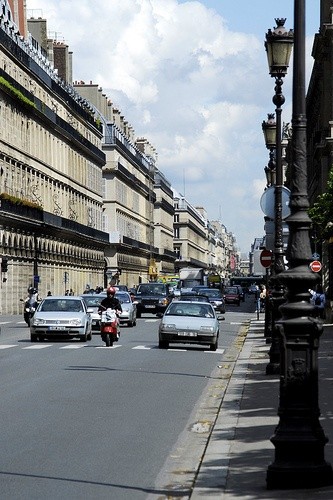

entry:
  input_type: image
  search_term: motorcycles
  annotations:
[96,301,121,347]
[20,298,40,327]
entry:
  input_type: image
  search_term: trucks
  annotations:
[177,268,207,295]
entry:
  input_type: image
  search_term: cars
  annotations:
[156,301,225,351]
[30,296,92,342]
[233,285,245,302]
[176,295,210,310]
[221,287,240,307]
[192,286,208,292]
[99,292,139,327]
[198,289,225,314]
[80,294,107,326]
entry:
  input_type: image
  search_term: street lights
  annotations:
[262,113,283,344]
[265,18,294,374]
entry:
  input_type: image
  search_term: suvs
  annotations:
[133,283,174,318]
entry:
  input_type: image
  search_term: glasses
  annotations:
[108,292,113,295]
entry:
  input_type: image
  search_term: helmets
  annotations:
[106,287,115,298]
[27,287,34,294]
[34,288,37,293]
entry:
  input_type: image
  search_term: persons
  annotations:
[21,286,42,302]
[97,287,122,339]
[260,284,267,312]
[126,293,135,302]
[65,290,69,295]
[70,289,75,296]
[255,286,261,312]
[85,285,103,294]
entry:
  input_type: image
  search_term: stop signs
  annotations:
[260,250,273,268]
[311,261,321,272]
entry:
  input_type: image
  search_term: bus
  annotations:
[230,277,263,295]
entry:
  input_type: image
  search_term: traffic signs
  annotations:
[264,221,289,234]
[260,186,291,220]
[265,234,289,249]
